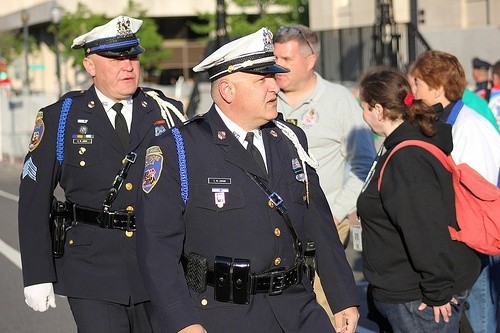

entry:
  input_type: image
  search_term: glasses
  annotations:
[278,26,320,55]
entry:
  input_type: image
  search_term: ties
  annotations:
[245,132,268,178]
[112,103,129,151]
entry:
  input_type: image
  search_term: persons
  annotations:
[270,21,500,333]
[136,28,361,333]
[17,15,187,333]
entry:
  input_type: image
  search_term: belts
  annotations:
[180,254,306,295]
[64,200,138,232]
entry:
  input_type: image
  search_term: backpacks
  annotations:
[377,139,500,256]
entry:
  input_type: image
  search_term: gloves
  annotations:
[23,283,56,312]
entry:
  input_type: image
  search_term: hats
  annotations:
[71,16,145,58]
[472,58,491,70]
[192,27,291,81]
